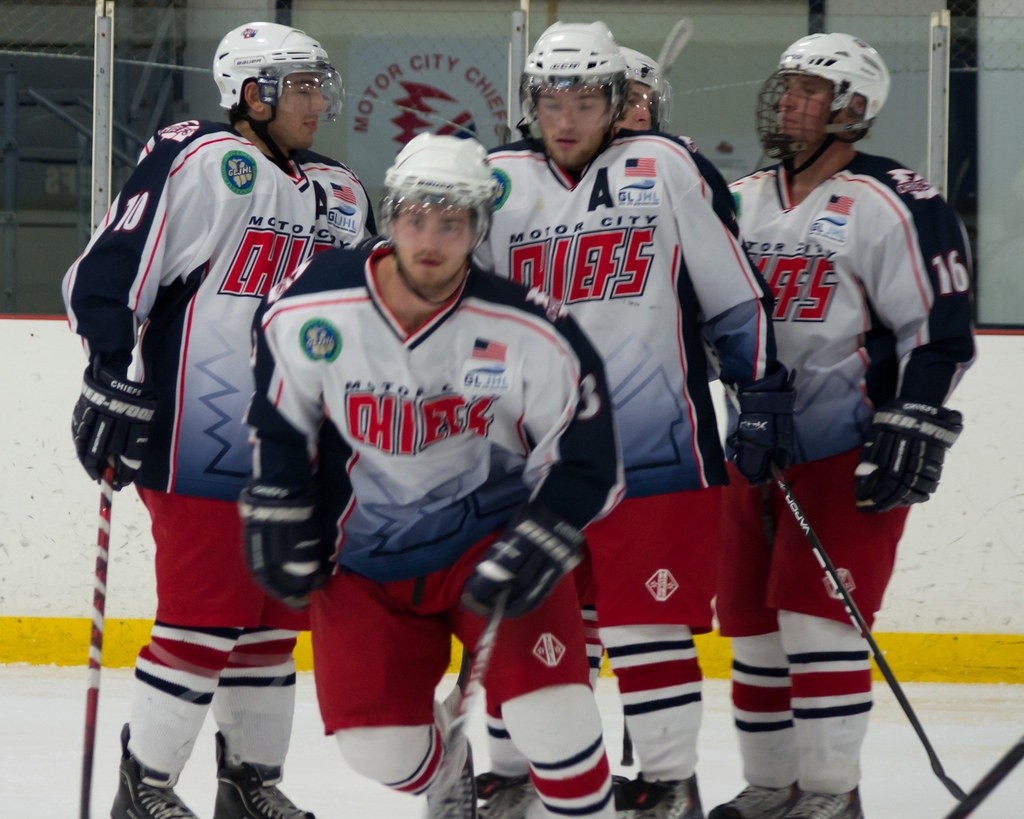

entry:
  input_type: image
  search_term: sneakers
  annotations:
[707,780,801,819]
[425,732,477,819]
[474,771,703,819]
[211,730,316,819]
[110,721,198,818]
[781,785,864,818]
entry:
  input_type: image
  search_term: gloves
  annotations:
[72,358,170,493]
[467,506,592,618]
[725,360,799,487]
[857,400,964,514]
[237,481,339,610]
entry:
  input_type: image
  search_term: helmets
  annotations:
[776,31,892,121]
[520,18,626,106]
[385,132,500,210]
[619,46,667,104]
[212,21,330,113]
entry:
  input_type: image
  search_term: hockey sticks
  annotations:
[75,455,119,819]
[943,738,1024,819]
[506,6,525,144]
[705,340,970,805]
[646,13,695,132]
[426,591,512,818]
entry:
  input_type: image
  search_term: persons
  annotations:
[471,21,798,819]
[708,33,976,819]
[60,22,378,819]
[239,131,625,819]
